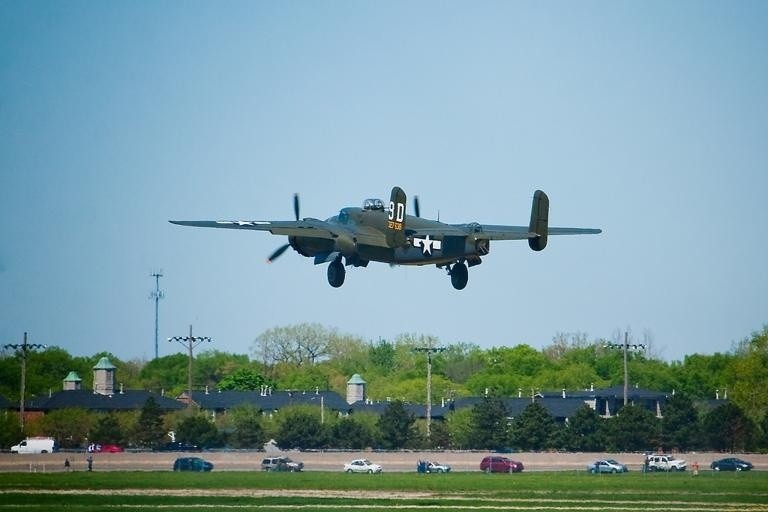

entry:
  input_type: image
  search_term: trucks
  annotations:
[10,435,57,456]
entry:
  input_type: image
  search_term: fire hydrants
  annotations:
[692,461,701,477]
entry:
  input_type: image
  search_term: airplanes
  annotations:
[167,184,604,291]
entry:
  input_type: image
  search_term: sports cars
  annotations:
[710,457,754,471]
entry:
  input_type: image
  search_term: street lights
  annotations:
[414,346,447,439]
[149,273,164,359]
[3,331,49,433]
[166,323,212,406]
[603,333,648,406]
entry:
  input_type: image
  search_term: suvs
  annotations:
[261,456,305,471]
[646,454,688,471]
[479,456,525,472]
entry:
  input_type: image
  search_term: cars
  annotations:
[173,457,214,471]
[343,458,383,474]
[89,444,124,453]
[588,459,625,473]
[427,460,451,474]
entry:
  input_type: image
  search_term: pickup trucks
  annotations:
[151,442,198,452]
